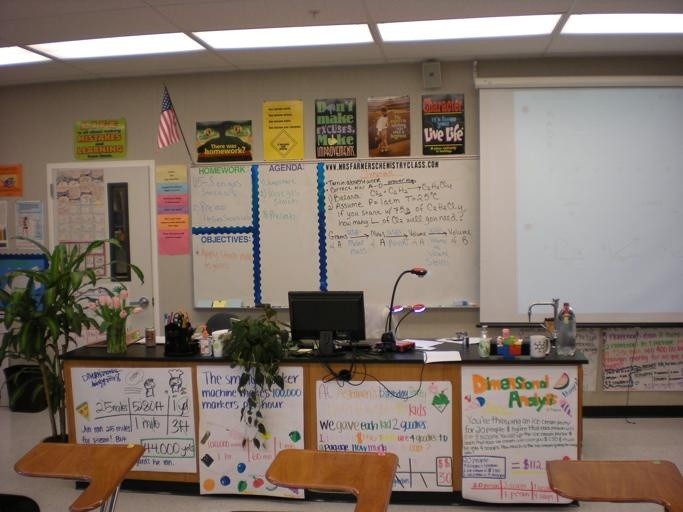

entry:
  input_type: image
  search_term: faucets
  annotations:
[526,298,560,330]
[456,331,469,349]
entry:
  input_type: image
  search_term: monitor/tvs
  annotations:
[287,290,366,355]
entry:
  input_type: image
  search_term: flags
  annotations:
[156,86,181,150]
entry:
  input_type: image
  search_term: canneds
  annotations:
[144,327,155,348]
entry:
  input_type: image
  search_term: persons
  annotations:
[376,106,390,149]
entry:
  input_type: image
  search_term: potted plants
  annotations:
[218,304,288,450]
[0,234,144,443]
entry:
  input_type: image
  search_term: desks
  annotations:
[13,443,146,512]
[545,458,683,512]
[58,337,588,506]
[264,443,399,512]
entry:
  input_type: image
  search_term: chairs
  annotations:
[0,493,40,512]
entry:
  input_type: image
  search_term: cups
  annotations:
[198,332,225,358]
[529,335,550,359]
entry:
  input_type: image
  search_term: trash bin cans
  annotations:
[4,364,48,413]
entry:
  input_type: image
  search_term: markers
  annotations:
[454,301,476,306]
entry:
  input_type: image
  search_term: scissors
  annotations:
[173,313,184,328]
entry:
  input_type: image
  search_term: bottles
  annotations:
[477,325,491,358]
[556,302,577,357]
[462,335,469,351]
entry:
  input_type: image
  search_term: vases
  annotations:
[106,321,128,354]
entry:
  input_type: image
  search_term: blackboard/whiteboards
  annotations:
[187,154,481,309]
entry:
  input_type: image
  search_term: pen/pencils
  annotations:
[163,305,192,344]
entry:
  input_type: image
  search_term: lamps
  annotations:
[374,267,428,353]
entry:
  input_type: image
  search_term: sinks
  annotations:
[486,340,531,356]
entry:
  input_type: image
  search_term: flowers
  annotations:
[85,288,145,354]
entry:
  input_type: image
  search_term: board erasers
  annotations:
[255,304,270,308]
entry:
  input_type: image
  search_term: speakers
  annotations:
[424,60,440,88]
[382,331,397,355]
